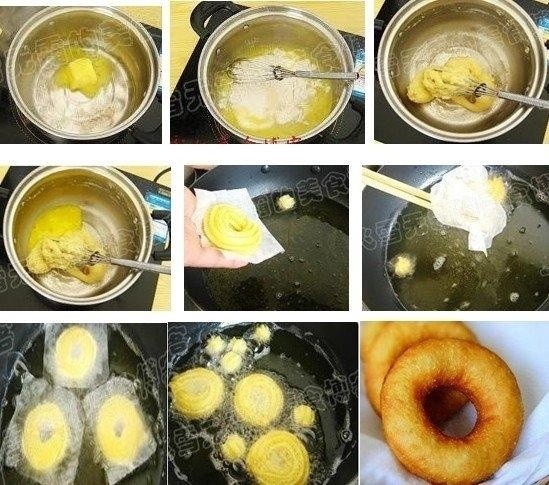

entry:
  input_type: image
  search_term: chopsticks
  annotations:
[363,165,432,213]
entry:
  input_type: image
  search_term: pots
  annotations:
[184,166,348,309]
[168,323,359,483]
[1,165,170,306]
[1,324,169,485]
[363,165,548,310]
[4,7,160,143]
[188,1,355,142]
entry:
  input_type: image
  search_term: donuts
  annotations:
[360,320,525,485]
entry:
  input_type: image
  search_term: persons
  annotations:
[184,186,249,268]
